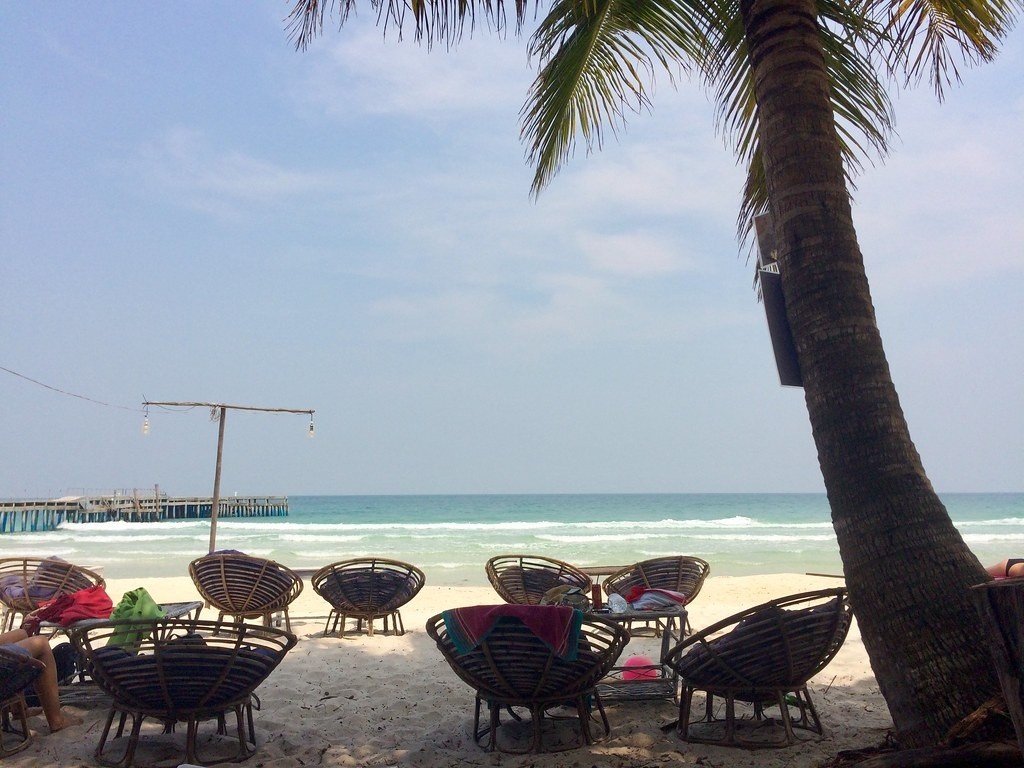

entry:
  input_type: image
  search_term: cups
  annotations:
[592,584,601,609]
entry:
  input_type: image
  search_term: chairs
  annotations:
[0,646,47,759]
[188,550,305,638]
[311,556,425,639]
[0,557,107,643]
[71,619,299,768]
[427,554,855,755]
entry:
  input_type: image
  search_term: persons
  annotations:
[1,629,84,733]
[985,560,1023,579]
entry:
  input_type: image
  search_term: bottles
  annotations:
[609,593,627,613]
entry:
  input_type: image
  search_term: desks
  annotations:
[37,600,204,687]
[576,602,688,706]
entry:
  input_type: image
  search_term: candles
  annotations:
[592,574,603,609]
